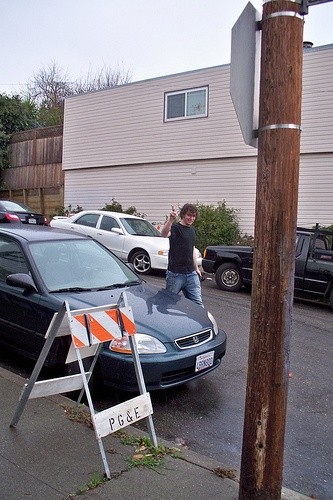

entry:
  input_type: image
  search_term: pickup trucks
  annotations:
[202,222,333,308]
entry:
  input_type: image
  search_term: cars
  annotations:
[0,224,227,400]
[49,210,203,276]
[0,200,48,226]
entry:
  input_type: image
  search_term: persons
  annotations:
[161,203,205,310]
